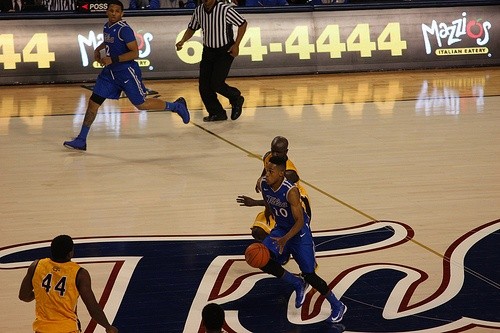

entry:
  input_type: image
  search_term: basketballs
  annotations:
[245,243,270,268]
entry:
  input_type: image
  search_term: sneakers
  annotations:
[294,277,313,308]
[64,137,86,151]
[331,301,347,323]
[230,96,244,120]
[203,110,228,122]
[172,97,189,124]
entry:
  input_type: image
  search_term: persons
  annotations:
[176,0,247,123]
[259,156,347,322]
[250,136,312,241]
[63,0,190,152]
[202,303,226,333]
[19,235,118,333]
